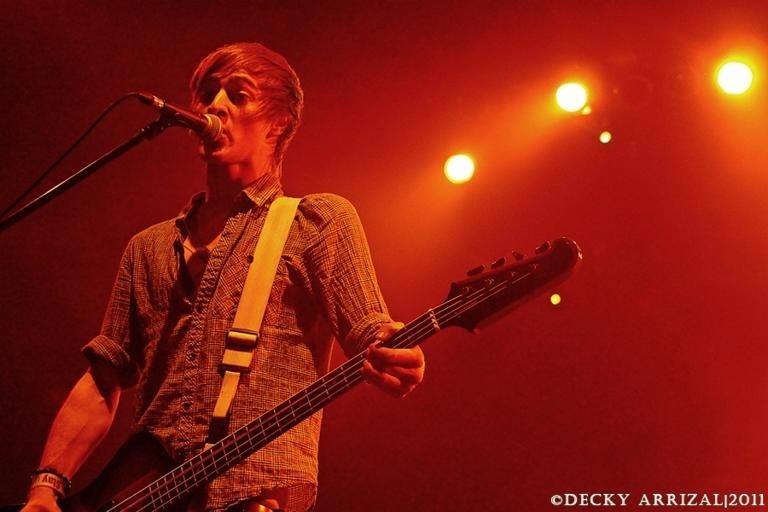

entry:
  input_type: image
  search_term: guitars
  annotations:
[57,234,577,510]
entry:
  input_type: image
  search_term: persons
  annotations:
[14,42,427,511]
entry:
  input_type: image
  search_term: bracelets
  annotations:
[26,468,71,494]
[29,472,64,496]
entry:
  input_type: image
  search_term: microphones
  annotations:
[137,91,222,142]
